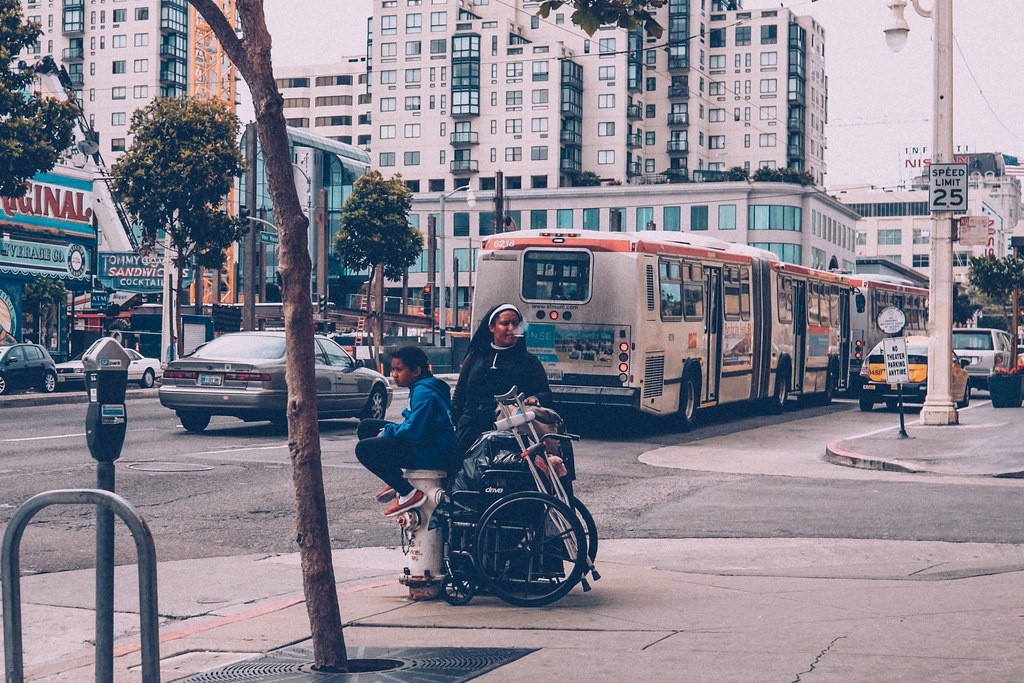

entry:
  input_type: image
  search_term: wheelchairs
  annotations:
[439,408,602,610]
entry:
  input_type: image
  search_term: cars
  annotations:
[49,346,163,390]
[0,344,58,395]
[157,330,395,442]
[857,333,972,412]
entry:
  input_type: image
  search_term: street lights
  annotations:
[439,182,477,348]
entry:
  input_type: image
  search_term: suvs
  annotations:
[949,328,1016,384]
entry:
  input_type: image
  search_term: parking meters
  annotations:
[78,336,135,682]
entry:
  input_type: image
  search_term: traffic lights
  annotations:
[422,283,433,317]
[240,205,251,233]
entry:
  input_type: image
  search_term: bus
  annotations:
[828,272,932,399]
[470,225,866,434]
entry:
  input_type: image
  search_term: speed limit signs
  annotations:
[929,164,967,212]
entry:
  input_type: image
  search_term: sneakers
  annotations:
[376,484,396,503]
[383,489,428,517]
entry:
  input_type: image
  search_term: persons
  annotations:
[25,335,36,356]
[354,346,459,517]
[450,300,555,467]
[165,336,178,365]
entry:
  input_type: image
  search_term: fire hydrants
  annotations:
[394,466,451,601]
